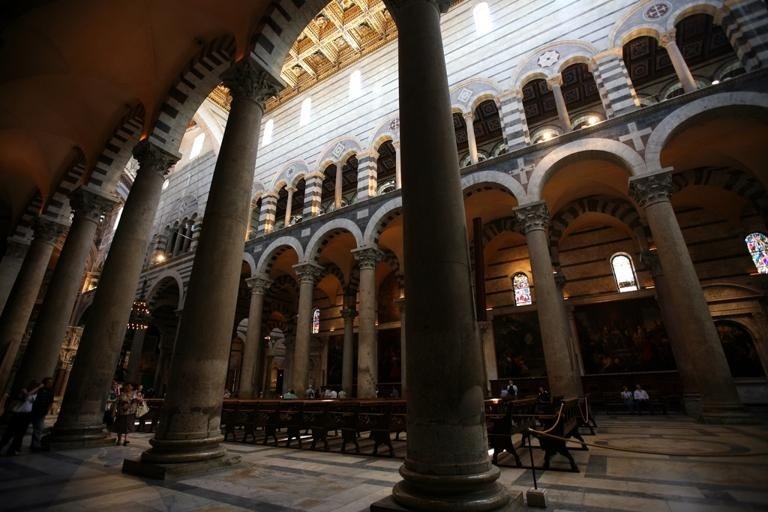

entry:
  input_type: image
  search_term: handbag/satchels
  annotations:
[134,400,150,418]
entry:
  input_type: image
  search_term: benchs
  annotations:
[603,390,669,415]
[104,395,598,472]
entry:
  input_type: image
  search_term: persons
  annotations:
[283,385,349,400]
[538,388,548,402]
[102,380,148,446]
[500,380,518,397]
[2,376,54,454]
[620,384,635,414]
[633,384,656,416]
[223,389,231,399]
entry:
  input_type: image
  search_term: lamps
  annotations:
[126,165,175,331]
